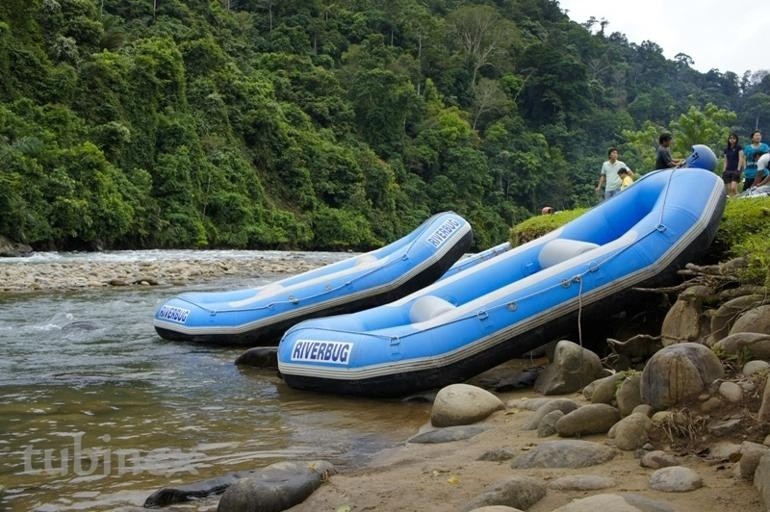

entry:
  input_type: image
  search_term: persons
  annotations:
[596,148,634,200]
[722,131,770,197]
[656,133,684,169]
[618,168,633,191]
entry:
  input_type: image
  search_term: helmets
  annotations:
[687,144,717,172]
